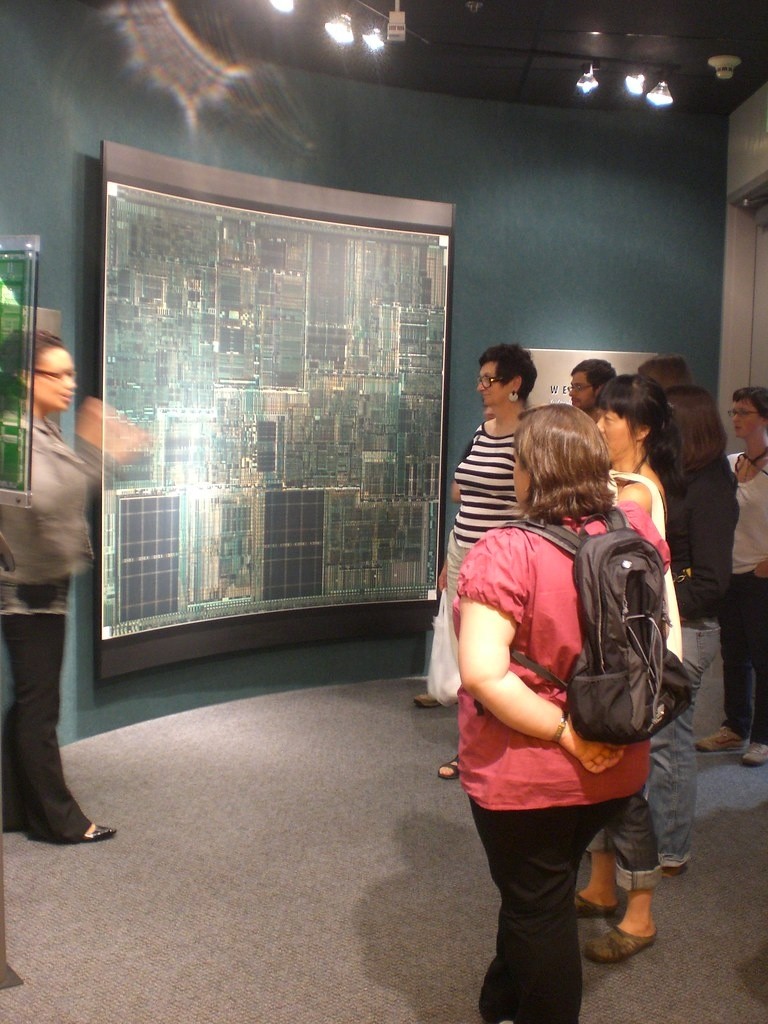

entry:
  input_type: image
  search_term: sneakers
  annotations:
[742,741,768,765]
[416,695,458,708]
[695,725,745,750]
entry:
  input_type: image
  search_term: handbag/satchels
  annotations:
[427,591,463,708]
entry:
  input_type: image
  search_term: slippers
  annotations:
[583,923,657,960]
[575,892,620,919]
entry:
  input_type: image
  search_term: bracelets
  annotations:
[553,711,567,743]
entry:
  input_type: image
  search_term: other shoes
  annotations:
[660,863,685,878]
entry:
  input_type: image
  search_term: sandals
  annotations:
[436,755,460,778]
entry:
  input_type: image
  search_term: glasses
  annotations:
[475,375,504,386]
[567,384,593,392]
[727,409,760,419]
[29,367,80,381]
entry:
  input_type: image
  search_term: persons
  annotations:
[415,393,495,708]
[450,405,672,1024]
[0,328,151,847]
[435,342,537,781]
[567,352,768,962]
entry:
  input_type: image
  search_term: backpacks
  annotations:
[496,508,690,744]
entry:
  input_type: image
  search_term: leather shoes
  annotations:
[82,825,116,841]
